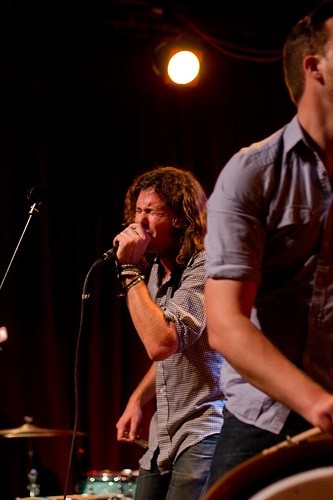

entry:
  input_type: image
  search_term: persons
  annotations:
[200,9,333,500]
[112,166,227,500]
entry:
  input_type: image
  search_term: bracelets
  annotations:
[116,263,145,297]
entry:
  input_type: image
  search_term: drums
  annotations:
[84,469,137,500]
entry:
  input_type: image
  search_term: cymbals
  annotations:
[0,424,84,438]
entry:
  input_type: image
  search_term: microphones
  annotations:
[100,241,119,261]
[26,183,47,202]
